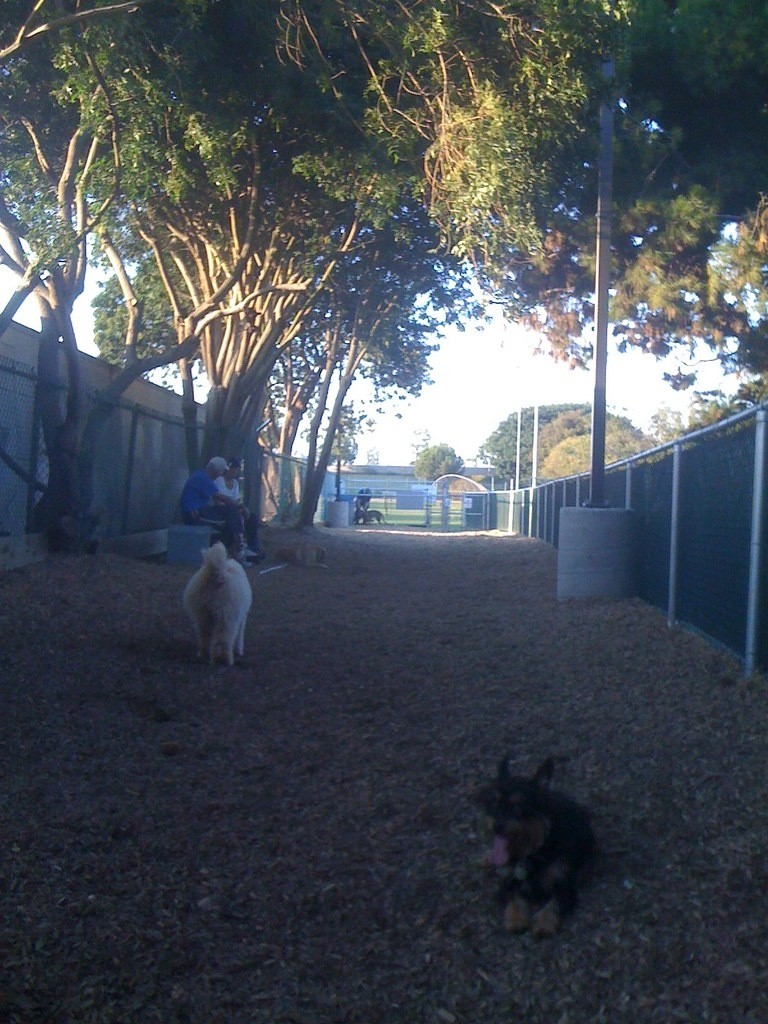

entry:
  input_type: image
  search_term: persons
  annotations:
[180,456,257,569]
[212,459,265,564]
[355,488,372,524]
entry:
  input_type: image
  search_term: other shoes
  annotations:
[239,548,258,557]
[239,561,254,569]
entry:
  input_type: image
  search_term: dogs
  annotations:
[233,533,258,568]
[483,755,597,929]
[183,541,252,666]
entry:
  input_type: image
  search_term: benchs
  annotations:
[165,523,221,567]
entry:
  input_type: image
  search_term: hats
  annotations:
[211,456,230,473]
